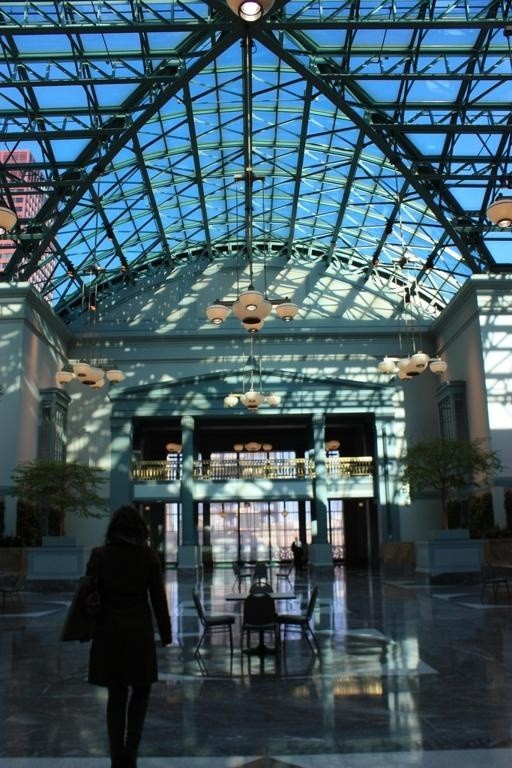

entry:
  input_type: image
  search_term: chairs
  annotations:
[1,575,25,608]
[190,558,321,658]
[478,560,509,602]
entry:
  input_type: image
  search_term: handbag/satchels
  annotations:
[63,591,101,642]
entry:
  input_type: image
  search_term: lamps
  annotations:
[377,163,448,381]
[56,140,127,392]
[485,194,512,230]
[200,166,302,409]
[0,199,17,239]
[226,0,274,24]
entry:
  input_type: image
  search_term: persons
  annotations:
[291,537,305,570]
[84,505,173,768]
